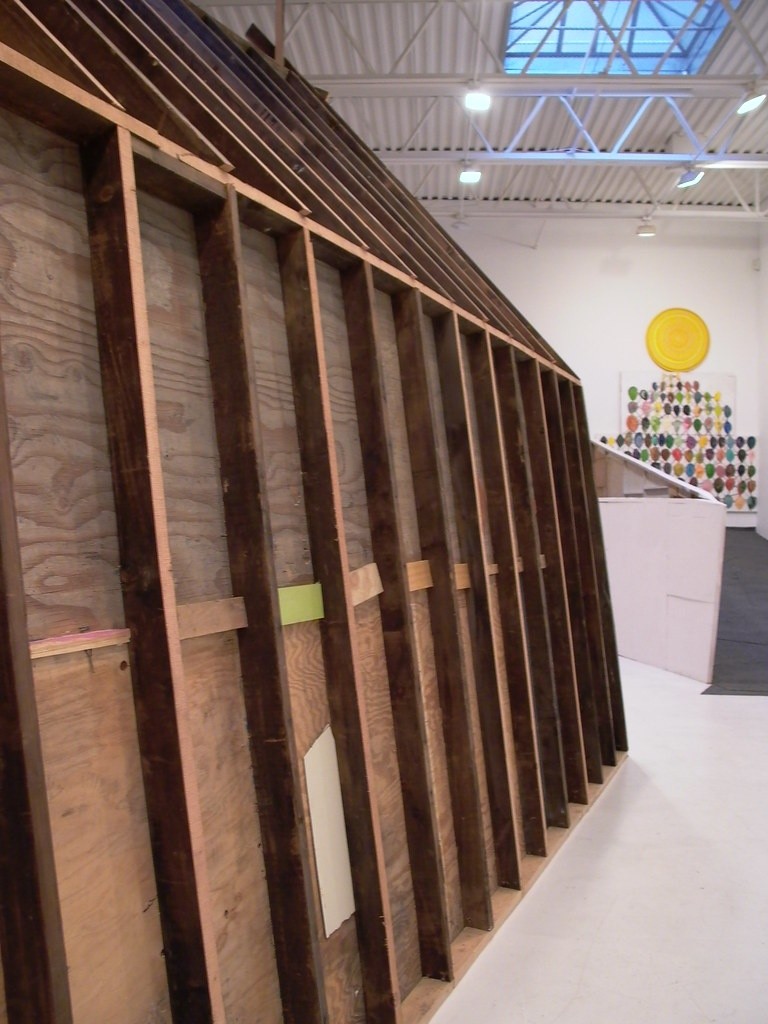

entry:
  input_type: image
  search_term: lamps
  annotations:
[465,82,490,112]
[677,171,704,189]
[737,92,767,115]
[460,171,481,184]
[636,225,656,236]
